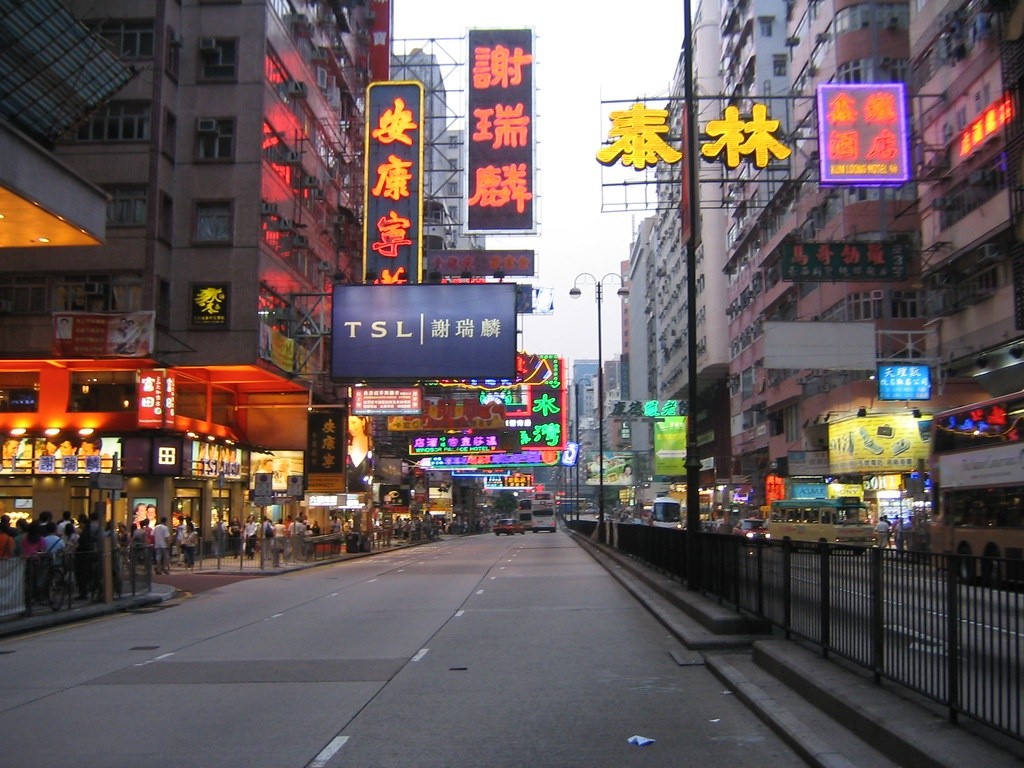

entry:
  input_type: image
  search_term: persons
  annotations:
[56,318,71,338]
[394,511,443,542]
[618,464,633,485]
[113,318,140,354]
[0,511,105,608]
[874,514,905,556]
[590,455,608,472]
[328,516,351,553]
[214,510,321,565]
[107,516,198,597]
[132,503,157,529]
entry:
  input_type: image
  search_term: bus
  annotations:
[531,491,556,533]
[641,506,652,525]
[650,496,681,530]
[767,498,876,554]
[930,390,1024,586]
[518,499,532,530]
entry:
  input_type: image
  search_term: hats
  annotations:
[882,515,887,519]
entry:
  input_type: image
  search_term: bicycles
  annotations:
[46,550,75,611]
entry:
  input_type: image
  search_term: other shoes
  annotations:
[74,595,84,600]
[155,568,160,575]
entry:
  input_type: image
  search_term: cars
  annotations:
[493,519,525,535]
[586,507,634,521]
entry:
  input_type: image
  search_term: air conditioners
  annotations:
[932,196,952,211]
[197,118,220,136]
[816,384,829,393]
[199,37,216,54]
[871,290,883,301]
[975,244,1007,263]
[891,290,905,300]
[83,282,104,296]
[795,377,806,386]
[928,273,953,291]
[261,0,376,272]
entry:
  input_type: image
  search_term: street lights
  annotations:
[568,273,629,540]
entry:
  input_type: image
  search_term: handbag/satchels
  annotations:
[64,571,77,585]
[170,545,178,556]
[265,521,272,537]
[232,529,241,537]
[243,542,247,551]
[180,544,185,548]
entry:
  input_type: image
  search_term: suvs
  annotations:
[731,518,768,544]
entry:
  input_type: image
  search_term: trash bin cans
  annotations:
[346,532,361,553]
[361,531,373,552]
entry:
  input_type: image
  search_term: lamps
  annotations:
[802,418,825,428]
[857,406,867,417]
[912,407,923,418]
[823,410,850,422]
[812,414,839,425]
[1008,347,1024,358]
